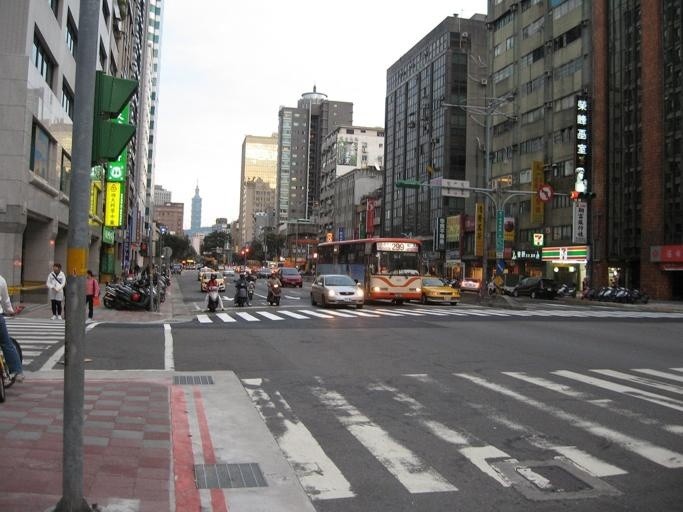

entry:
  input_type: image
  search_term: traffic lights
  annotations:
[569,192,597,200]
[92,68,139,168]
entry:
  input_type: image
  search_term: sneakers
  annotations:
[86,318,93,322]
[50,314,62,321]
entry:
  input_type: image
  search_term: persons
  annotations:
[234,271,255,306]
[575,167,588,194]
[0,275,26,383]
[85,270,100,324]
[202,273,225,312]
[46,263,66,321]
[267,273,282,302]
[124,263,155,280]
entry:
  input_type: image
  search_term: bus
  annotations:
[186,260,196,269]
[316,238,422,304]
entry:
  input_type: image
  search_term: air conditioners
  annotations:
[481,79,488,84]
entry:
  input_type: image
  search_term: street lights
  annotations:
[241,248,249,267]
[434,87,519,295]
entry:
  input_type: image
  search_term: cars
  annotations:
[256,266,364,308]
[422,277,556,306]
[171,264,181,275]
[199,267,235,292]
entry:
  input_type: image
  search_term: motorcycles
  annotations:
[207,281,255,312]
[557,284,649,304]
[104,265,170,311]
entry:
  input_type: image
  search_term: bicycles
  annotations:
[0,312,23,402]
